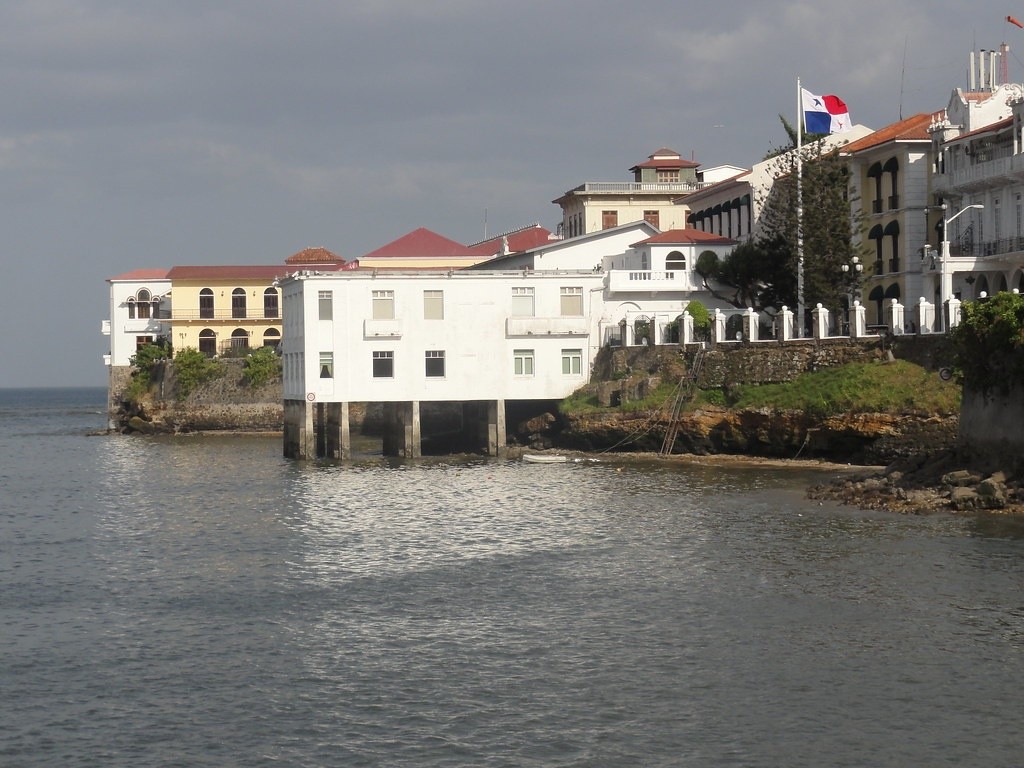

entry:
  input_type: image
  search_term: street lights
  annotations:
[841,257,864,305]
[942,204,985,334]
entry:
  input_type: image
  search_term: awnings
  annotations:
[711,204,721,215]
[868,285,884,301]
[729,198,741,209]
[882,157,898,172]
[687,213,696,223]
[740,194,751,206]
[868,223,883,240]
[883,219,899,235]
[884,283,900,298]
[867,162,882,177]
[704,207,712,218]
[694,210,704,221]
[721,201,730,213]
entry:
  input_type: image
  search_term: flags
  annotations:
[801,88,853,134]
